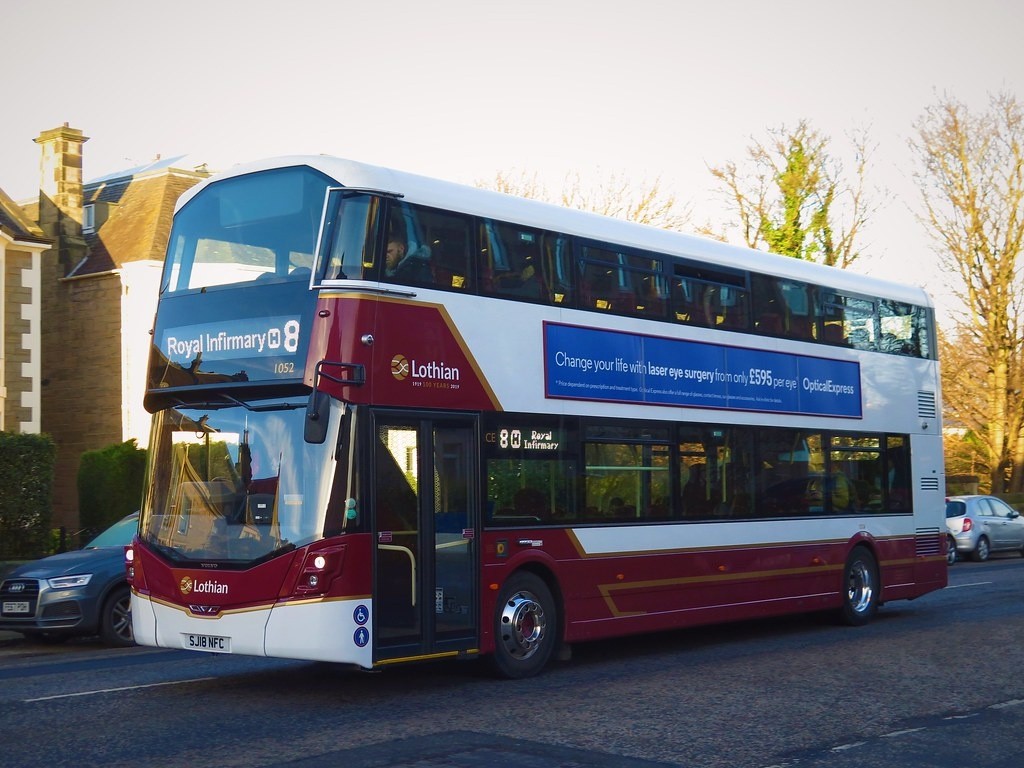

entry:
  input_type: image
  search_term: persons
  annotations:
[866,459,903,505]
[682,462,734,517]
[804,459,849,508]
[609,497,626,512]
[384,235,435,283]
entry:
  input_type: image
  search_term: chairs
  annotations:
[430,262,845,345]
[511,489,549,525]
[889,488,910,510]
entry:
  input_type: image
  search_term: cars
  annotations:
[945,495,1024,566]
[0,510,140,648]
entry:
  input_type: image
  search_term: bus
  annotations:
[123,154,951,683]
